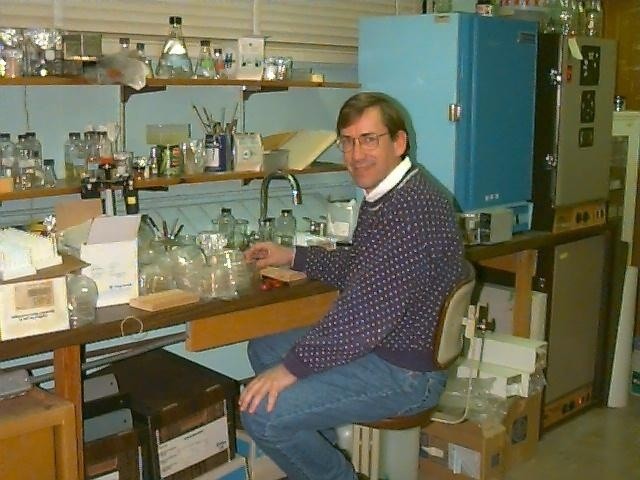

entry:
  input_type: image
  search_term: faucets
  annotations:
[257,169,303,230]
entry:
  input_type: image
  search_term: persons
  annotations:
[236,90,468,480]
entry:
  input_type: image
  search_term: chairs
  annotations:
[353,260,477,480]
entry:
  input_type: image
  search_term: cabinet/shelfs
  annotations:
[0,77,362,202]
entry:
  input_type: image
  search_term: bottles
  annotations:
[219,206,295,249]
[263,56,292,80]
[0,131,133,193]
[118,16,233,81]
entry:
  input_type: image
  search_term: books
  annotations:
[130,286,199,315]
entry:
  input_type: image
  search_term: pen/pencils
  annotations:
[193,102,238,134]
[141,214,184,251]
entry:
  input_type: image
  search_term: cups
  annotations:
[202,134,233,172]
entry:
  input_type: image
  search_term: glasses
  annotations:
[336,132,390,153]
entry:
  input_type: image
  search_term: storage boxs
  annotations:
[419,330,548,480]
[0,198,144,341]
[82,349,288,479]
